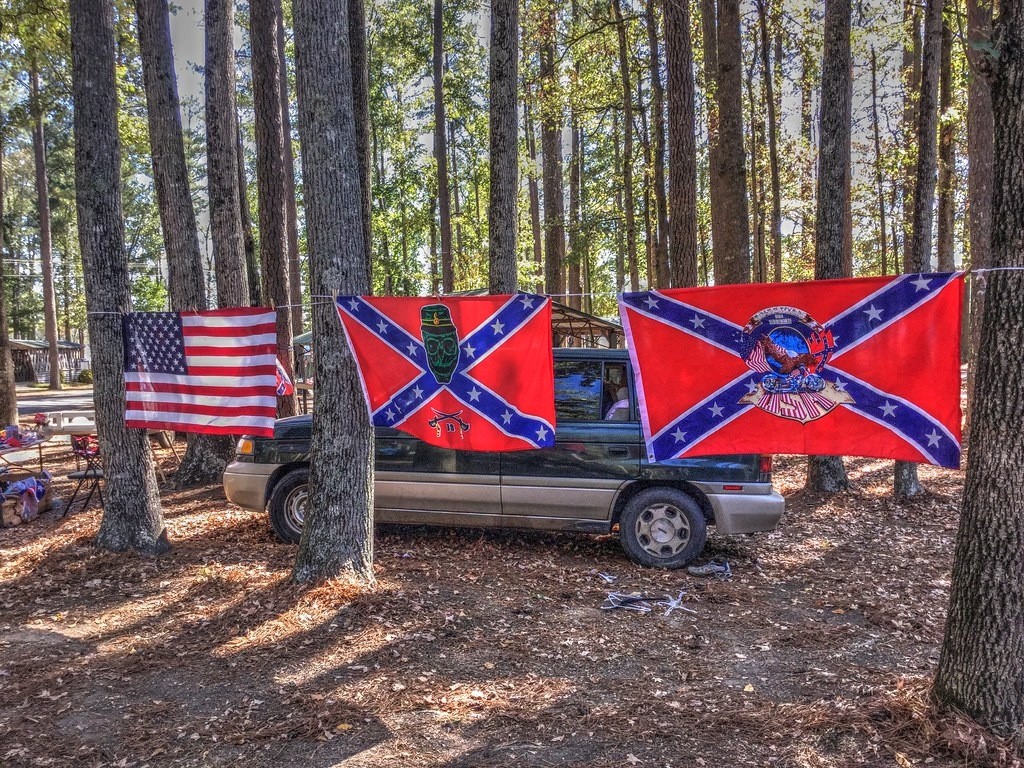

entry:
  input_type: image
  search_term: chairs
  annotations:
[62,446,105,519]
[606,387,630,421]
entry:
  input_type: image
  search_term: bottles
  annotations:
[24,424,45,439]
[21,500,32,523]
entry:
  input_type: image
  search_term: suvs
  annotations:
[221,347,787,572]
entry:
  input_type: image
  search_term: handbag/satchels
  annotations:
[0,468,53,527]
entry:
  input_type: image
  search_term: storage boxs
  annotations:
[0,474,53,514]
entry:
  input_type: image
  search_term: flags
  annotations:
[120,307,280,439]
[333,293,555,452]
[276,359,293,397]
[616,270,962,470]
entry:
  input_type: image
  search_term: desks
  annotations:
[0,424,53,474]
[40,412,181,484]
